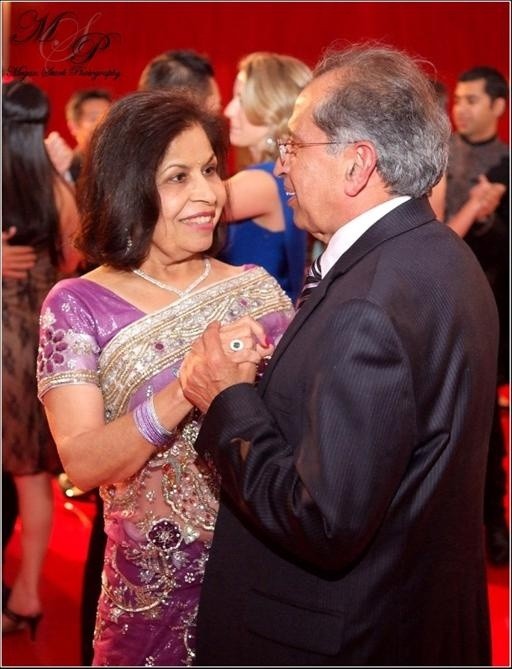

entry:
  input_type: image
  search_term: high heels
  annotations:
[2,605,43,641]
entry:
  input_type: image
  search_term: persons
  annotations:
[173,46,498,668]
[33,87,302,668]
[4,44,509,640]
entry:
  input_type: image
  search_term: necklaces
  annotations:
[126,255,213,298]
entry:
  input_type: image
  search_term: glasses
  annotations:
[276,137,376,167]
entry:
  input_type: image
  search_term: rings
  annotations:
[229,340,243,352]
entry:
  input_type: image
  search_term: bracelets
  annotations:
[131,395,179,447]
